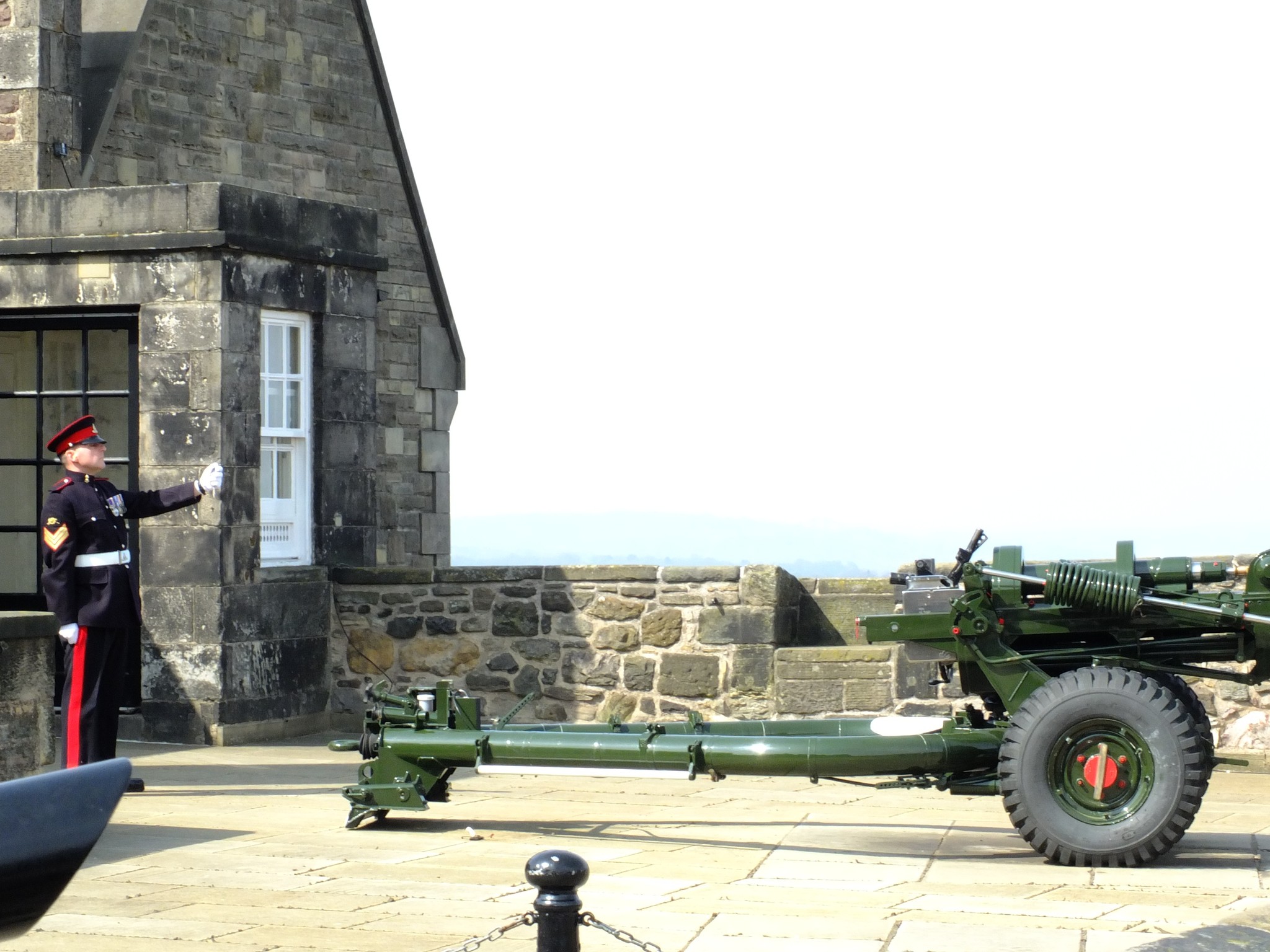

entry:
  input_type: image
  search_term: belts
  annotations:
[75,549,131,567]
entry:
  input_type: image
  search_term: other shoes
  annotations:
[125,778,145,792]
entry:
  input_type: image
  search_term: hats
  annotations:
[45,415,107,456]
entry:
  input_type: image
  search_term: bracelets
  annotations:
[198,478,205,495]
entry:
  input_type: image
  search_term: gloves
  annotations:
[194,462,223,498]
[58,623,79,644]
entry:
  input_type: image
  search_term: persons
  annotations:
[40,415,223,792]
